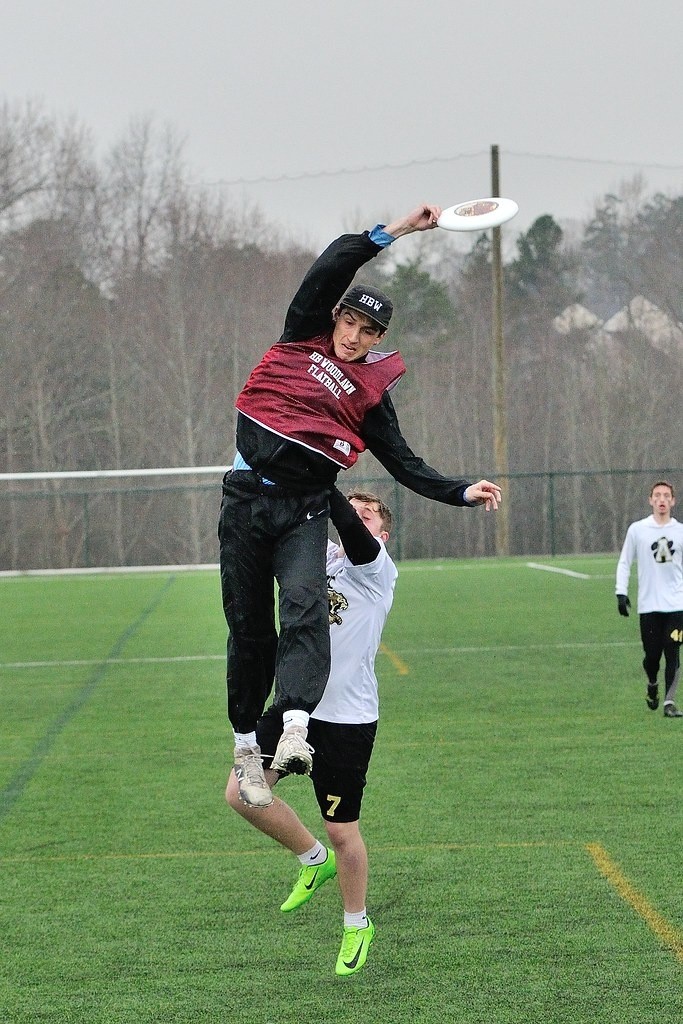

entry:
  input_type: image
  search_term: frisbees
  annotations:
[436,197,520,232]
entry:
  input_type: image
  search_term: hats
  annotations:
[341,284,394,329]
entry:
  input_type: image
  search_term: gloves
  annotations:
[616,595,631,617]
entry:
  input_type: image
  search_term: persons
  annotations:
[212,205,504,810]
[224,482,397,975]
[615,481,683,717]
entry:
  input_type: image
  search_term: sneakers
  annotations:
[234,744,275,809]
[269,724,315,777]
[646,684,660,710]
[663,700,683,717]
[280,847,337,913]
[335,915,375,976]
